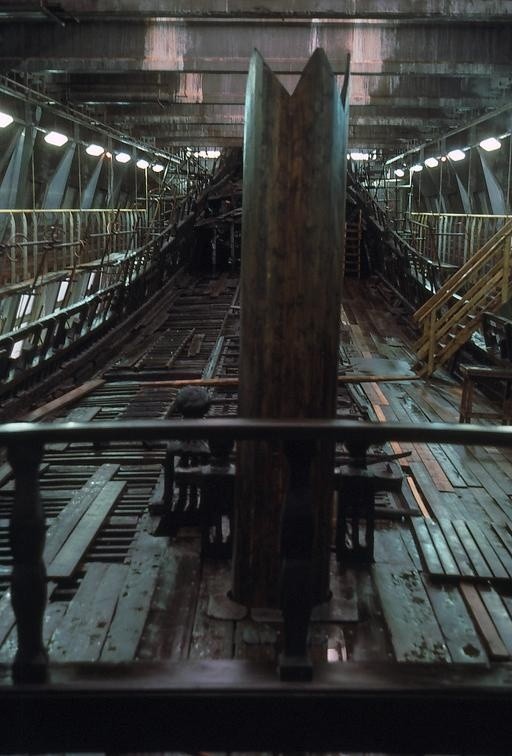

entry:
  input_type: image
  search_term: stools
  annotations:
[459,363,512,426]
